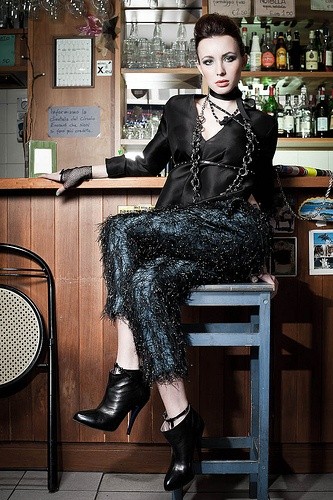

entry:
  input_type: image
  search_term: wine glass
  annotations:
[0,0,112,27]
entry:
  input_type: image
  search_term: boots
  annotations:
[160,405,205,493]
[72,363,151,434]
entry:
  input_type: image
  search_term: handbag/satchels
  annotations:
[274,164,333,223]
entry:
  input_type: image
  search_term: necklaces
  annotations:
[208,101,240,125]
[189,96,257,201]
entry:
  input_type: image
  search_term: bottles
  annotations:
[241,87,333,138]
[240,26,333,73]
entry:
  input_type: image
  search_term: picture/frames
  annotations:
[53,36,95,88]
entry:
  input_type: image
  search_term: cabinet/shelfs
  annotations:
[115,0,333,157]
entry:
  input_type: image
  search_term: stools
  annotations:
[172,282,273,500]
[0,243,63,494]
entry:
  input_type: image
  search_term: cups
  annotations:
[122,38,199,68]
[122,111,162,139]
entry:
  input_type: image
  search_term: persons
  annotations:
[37,13,282,491]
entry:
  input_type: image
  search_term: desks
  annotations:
[0,176,333,474]
